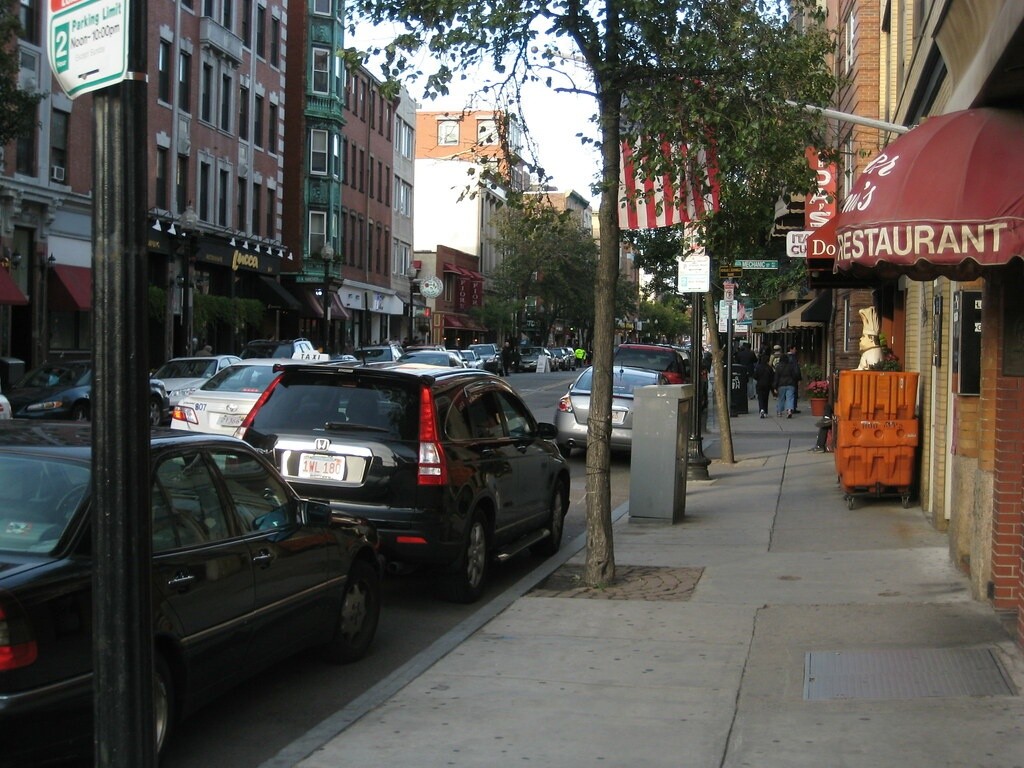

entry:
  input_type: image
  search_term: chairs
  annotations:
[347,389,377,425]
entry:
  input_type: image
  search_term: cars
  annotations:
[150,354,243,414]
[332,354,358,361]
[170,359,314,437]
[399,344,577,377]
[553,365,671,460]
[6,359,171,427]
[0,415,385,768]
[0,388,13,420]
[228,360,571,603]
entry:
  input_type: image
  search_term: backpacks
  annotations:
[771,355,780,372]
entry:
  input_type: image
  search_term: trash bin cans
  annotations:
[628,384,697,526]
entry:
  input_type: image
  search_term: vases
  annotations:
[810,398,828,417]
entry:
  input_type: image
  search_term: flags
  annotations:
[616,63,719,232]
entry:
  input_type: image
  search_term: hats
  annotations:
[774,345,782,349]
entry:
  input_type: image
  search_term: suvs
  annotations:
[355,342,404,365]
[239,338,319,361]
[613,342,708,413]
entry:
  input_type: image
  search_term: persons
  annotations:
[344,340,355,355]
[574,347,585,368]
[267,335,274,341]
[850,307,893,371]
[502,342,521,376]
[685,340,802,418]
[368,335,424,347]
[48,369,60,386]
[195,345,212,357]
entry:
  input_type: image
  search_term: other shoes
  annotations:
[760,409,767,419]
[776,412,783,416]
[808,445,824,453]
[815,417,831,427]
[786,412,792,419]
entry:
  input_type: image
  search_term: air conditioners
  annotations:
[51,165,64,181]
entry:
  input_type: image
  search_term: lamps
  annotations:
[285,246,293,260]
[148,207,161,232]
[11,247,22,270]
[161,211,176,236]
[272,240,283,258]
[225,227,235,247]
[47,253,56,274]
[249,234,260,253]
[262,236,272,256]
[237,231,248,250]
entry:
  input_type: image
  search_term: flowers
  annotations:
[804,380,829,397]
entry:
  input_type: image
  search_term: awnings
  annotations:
[49,266,95,313]
[763,286,833,338]
[835,108,1024,292]
[0,263,29,305]
[506,332,529,341]
[443,263,487,284]
[303,291,354,323]
[254,277,302,312]
[443,315,488,333]
[550,324,570,335]
[397,293,426,307]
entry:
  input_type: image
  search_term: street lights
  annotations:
[321,242,334,354]
[407,263,417,346]
